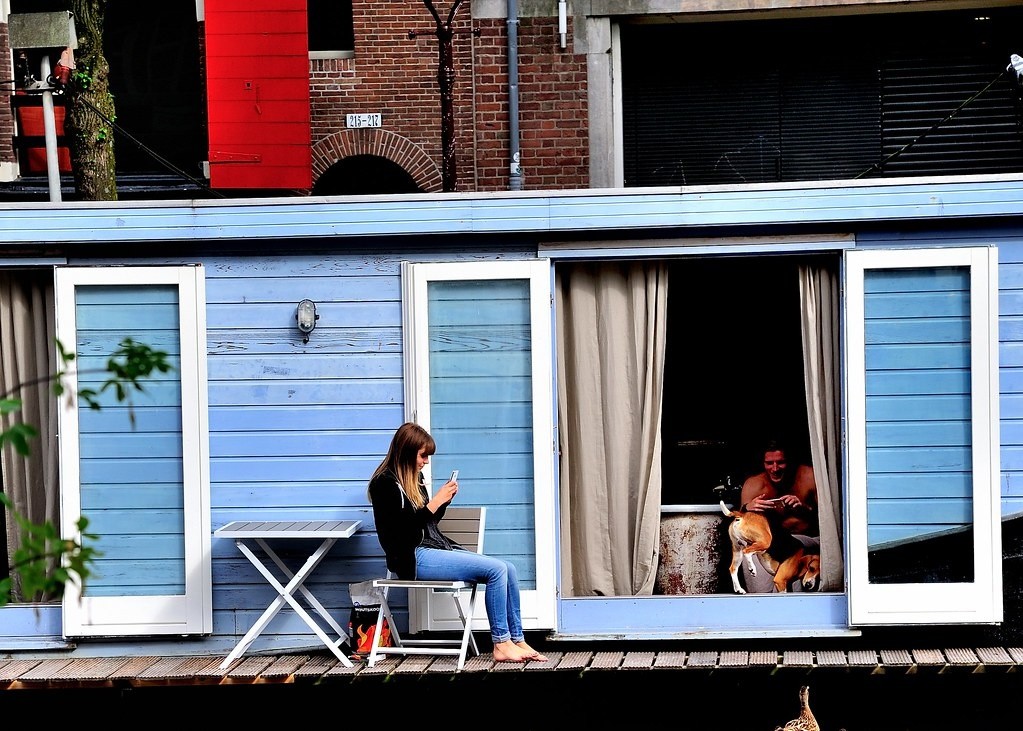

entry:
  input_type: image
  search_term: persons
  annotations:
[740,444,818,546]
[369,423,550,661]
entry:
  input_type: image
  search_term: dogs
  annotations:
[719,499,821,593]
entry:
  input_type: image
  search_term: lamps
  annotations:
[295,299,320,344]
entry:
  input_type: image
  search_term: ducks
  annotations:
[774,685,821,731]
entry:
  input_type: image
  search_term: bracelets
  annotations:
[743,502,749,512]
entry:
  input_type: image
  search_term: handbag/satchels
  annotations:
[348,602,394,663]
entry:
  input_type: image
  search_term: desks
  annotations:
[651,504,733,596]
[214,520,363,669]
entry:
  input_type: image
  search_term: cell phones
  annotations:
[450,471,459,482]
[767,499,785,508]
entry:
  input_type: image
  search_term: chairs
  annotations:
[367,507,486,669]
